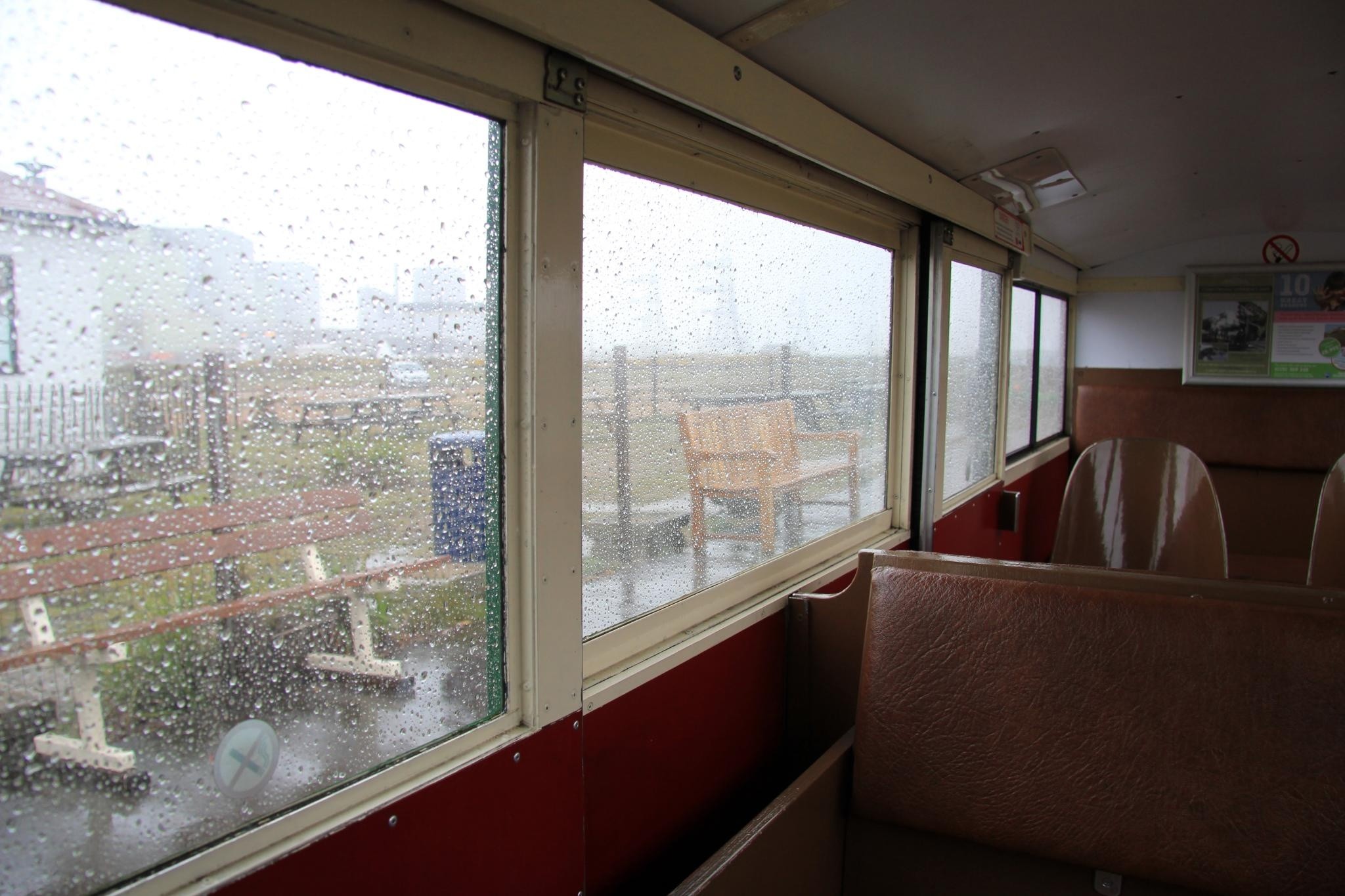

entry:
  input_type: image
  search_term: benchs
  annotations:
[680,399,859,552]
[0,484,452,794]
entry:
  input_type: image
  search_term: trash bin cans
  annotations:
[428,430,488,564]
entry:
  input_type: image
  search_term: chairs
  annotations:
[1306,454,1345,591]
[1049,437,1229,579]
